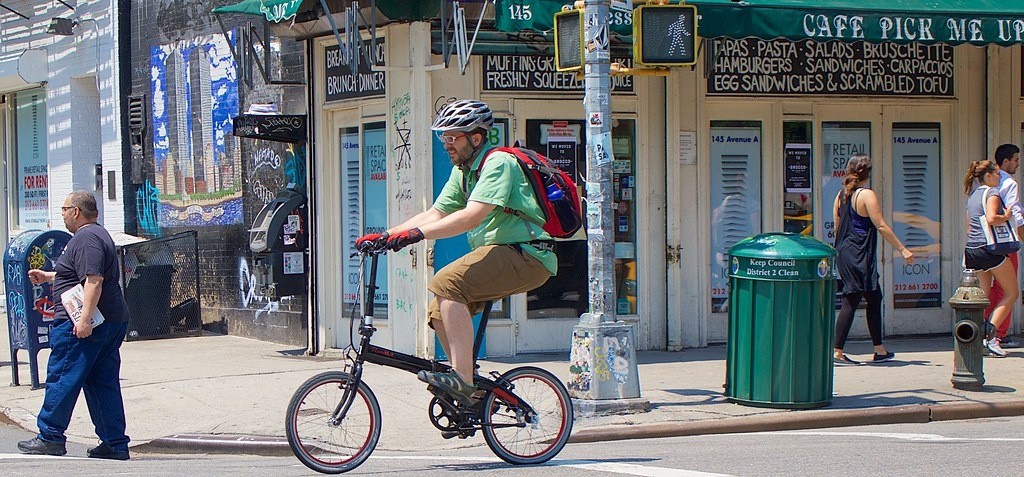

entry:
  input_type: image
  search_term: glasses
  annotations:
[440,132,472,143]
[62,206,81,213]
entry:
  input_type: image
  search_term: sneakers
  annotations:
[86,439,129,459]
[18,437,66,455]
[983,336,1007,356]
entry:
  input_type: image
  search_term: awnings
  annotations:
[213,0,440,23]
[494,0,1024,48]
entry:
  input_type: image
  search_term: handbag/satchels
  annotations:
[979,187,1021,255]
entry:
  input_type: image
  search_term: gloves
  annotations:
[354,231,389,249]
[386,228,424,252]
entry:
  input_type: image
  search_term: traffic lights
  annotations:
[553,9,585,71]
[633,5,697,66]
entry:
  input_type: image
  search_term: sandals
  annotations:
[418,367,478,407]
[441,418,479,439]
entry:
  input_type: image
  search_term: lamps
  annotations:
[46,17,78,35]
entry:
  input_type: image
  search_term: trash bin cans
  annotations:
[726,231,839,407]
[2,229,74,388]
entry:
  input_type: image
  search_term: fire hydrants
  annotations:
[947,268,991,389]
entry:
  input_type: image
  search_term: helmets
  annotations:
[432,100,493,132]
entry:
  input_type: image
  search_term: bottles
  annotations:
[547,182,563,200]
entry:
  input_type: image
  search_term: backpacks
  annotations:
[463,139,582,238]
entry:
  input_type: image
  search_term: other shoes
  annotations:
[874,351,894,362]
[1001,337,1020,348]
[833,355,860,366]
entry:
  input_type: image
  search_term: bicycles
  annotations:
[285,235,574,475]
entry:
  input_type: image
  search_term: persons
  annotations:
[984,144,1024,347]
[354,100,558,439]
[716,169,759,248]
[832,153,914,366]
[964,160,1019,355]
[18,191,131,460]
